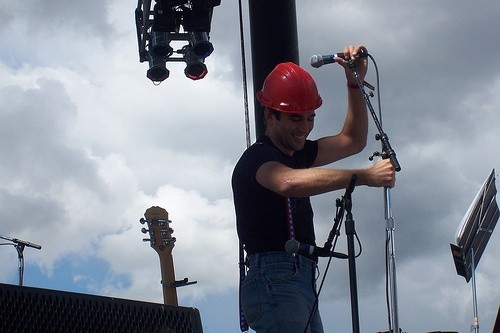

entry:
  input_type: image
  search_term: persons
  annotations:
[231,45,396,333]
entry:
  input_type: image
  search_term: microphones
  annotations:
[311,51,368,68]
[285,240,349,259]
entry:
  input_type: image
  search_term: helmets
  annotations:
[256,62,323,114]
